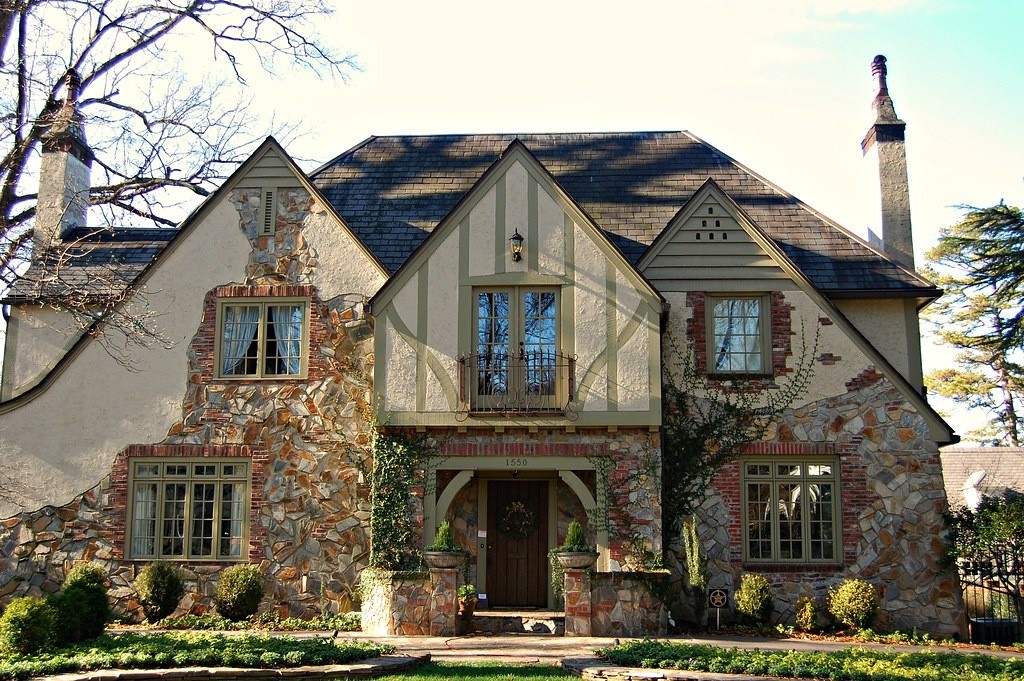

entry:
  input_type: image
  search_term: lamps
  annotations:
[510,470,520,478]
[509,228,524,262]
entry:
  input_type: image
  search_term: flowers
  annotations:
[496,495,541,540]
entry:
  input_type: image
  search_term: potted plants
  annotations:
[457,584,479,614]
[424,518,472,604]
[547,517,601,608]
